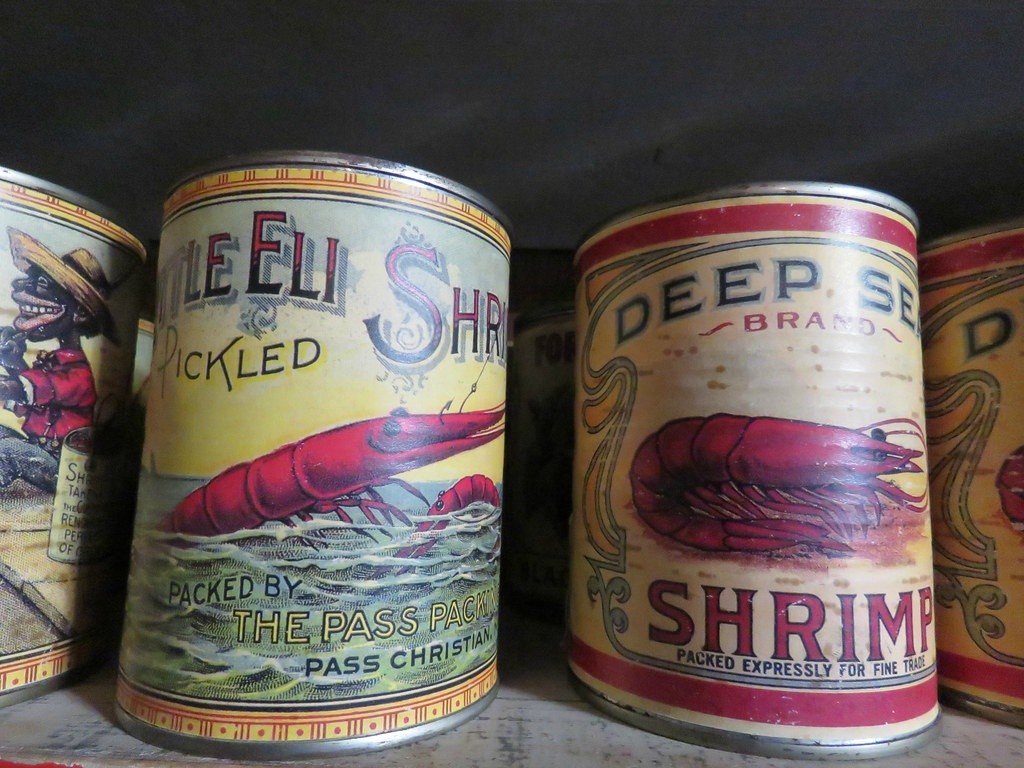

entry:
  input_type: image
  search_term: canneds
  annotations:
[0,149,1022,760]
